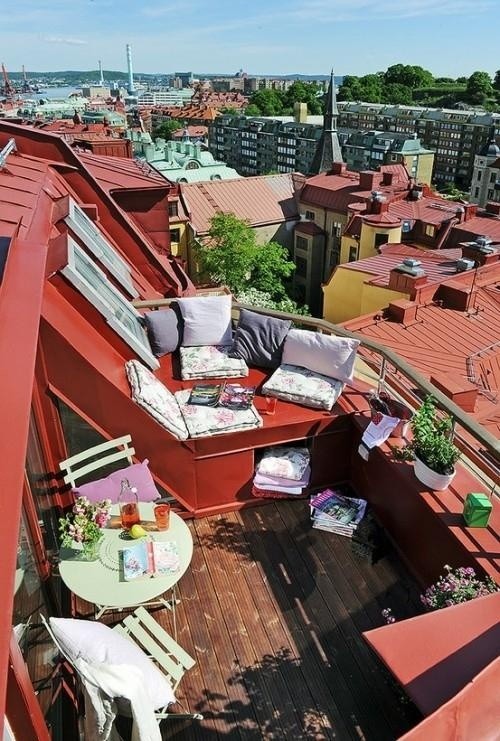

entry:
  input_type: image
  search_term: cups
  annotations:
[154,500,170,530]
[265,392,276,414]
[140,520,155,530]
[255,408,266,415]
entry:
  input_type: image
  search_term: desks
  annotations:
[58,503,195,640]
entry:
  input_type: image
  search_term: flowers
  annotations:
[60,495,113,563]
[381,565,499,622]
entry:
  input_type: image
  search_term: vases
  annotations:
[391,391,463,493]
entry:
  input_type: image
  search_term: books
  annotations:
[122,541,179,583]
[187,380,256,410]
[308,488,367,538]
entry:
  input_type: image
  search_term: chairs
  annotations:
[59,433,159,504]
[36,607,204,741]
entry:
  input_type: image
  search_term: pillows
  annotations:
[125,292,363,439]
[69,459,162,506]
[45,617,176,715]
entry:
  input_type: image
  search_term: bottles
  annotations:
[104,514,119,529]
[117,479,139,528]
